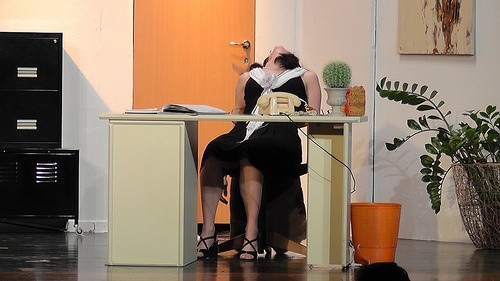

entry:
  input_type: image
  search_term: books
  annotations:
[122,104,230,114]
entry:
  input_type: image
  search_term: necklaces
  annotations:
[263,67,284,69]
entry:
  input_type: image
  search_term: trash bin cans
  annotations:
[350,202,402,265]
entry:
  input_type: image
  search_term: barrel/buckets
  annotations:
[351,202,402,265]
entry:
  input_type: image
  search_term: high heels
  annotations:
[240,237,258,261]
[196,231,218,259]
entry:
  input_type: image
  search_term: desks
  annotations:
[97,112,369,269]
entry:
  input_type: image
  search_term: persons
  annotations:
[197,45,321,261]
[353,262,411,281]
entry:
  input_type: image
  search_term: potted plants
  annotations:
[321,59,353,116]
[374,75,500,250]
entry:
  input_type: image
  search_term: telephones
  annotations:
[257,91,302,115]
